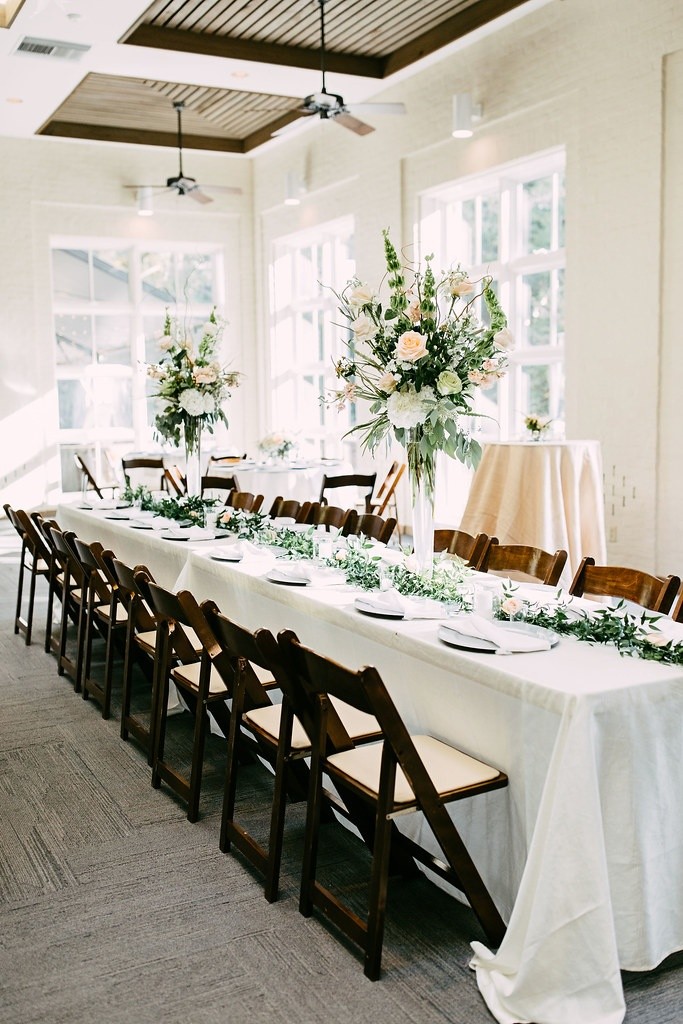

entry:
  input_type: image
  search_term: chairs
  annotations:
[3,453,683,981]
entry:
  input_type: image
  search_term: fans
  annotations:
[246,0,407,138]
[120,103,243,212]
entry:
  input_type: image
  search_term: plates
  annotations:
[160,528,230,539]
[228,453,341,473]
[265,570,311,584]
[354,595,404,617]
[78,504,92,509]
[129,519,152,529]
[210,544,289,561]
[437,620,560,651]
[105,514,129,519]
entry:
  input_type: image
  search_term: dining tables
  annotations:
[55,487,683,1024]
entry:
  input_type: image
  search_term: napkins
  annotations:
[132,516,179,528]
[162,526,216,540]
[103,508,146,519]
[272,560,346,585]
[357,588,445,620]
[214,540,276,560]
[439,613,551,652]
[77,497,118,510]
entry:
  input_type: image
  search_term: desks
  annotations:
[447,439,612,605]
[209,457,358,519]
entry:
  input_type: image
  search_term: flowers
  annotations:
[524,414,554,431]
[314,218,505,506]
[259,426,301,456]
[494,594,527,618]
[138,302,250,456]
[173,499,242,528]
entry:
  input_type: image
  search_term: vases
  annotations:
[183,425,201,502]
[403,426,436,580]
[532,429,540,440]
